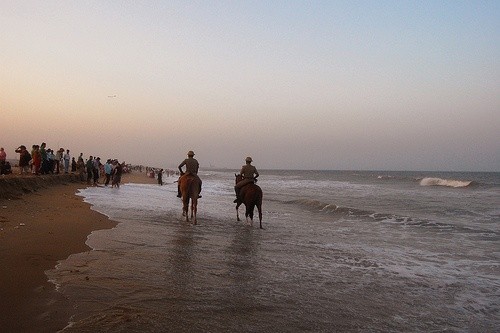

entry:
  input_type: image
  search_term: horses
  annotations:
[177,171,203,226]
[233,172,265,230]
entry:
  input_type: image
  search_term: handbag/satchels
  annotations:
[27,154,32,162]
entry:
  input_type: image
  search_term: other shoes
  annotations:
[177,194,181,198]
[198,196,202,198]
[234,199,237,203]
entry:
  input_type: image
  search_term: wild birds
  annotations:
[107,95,117,98]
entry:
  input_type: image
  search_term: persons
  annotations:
[30,143,64,176]
[103,159,123,188]
[176,151,202,198]
[64,150,70,173]
[122,163,158,177]
[158,169,163,186]
[85,156,101,187]
[77,153,84,181]
[15,145,30,174]
[0,148,11,175]
[234,157,259,203]
[72,157,77,171]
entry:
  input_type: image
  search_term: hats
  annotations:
[187,151,195,155]
[246,157,252,162]
